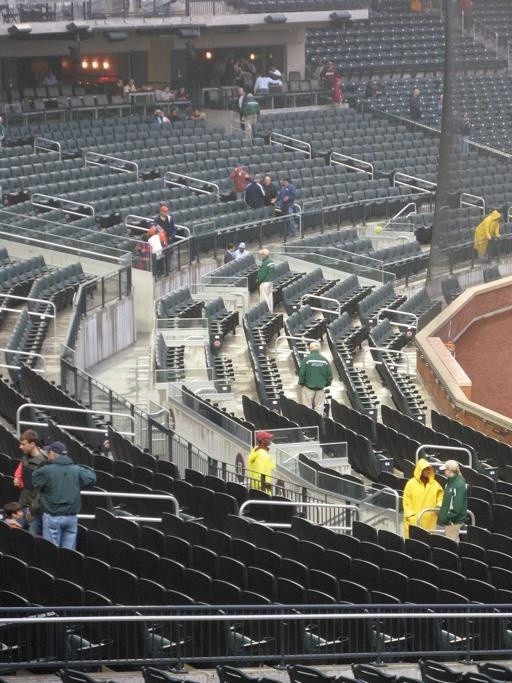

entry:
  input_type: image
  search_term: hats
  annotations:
[439,460,459,471]
[43,441,67,454]
[256,432,273,441]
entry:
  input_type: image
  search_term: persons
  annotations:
[41,68,58,86]
[463,115,471,136]
[298,342,333,416]
[247,431,275,496]
[3,502,24,530]
[100,436,115,462]
[154,205,177,272]
[367,82,380,97]
[320,61,348,108]
[230,164,296,237]
[409,89,423,119]
[147,225,163,282]
[111,56,283,125]
[470,209,501,270]
[14,429,46,536]
[224,243,236,264]
[31,441,96,552]
[402,458,444,539]
[234,242,248,260]
[436,94,444,115]
[437,460,467,547]
[255,249,274,315]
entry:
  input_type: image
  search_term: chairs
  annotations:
[0,1,512,678]
